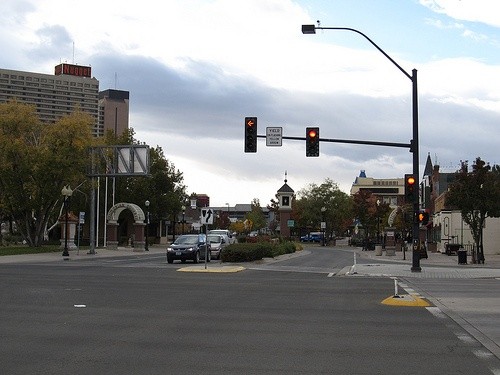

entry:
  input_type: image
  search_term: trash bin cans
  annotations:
[375,245,382,256]
[457,250,467,264]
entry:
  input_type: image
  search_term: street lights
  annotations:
[182,205,186,234]
[145,200,150,251]
[60,186,73,256]
[375,199,381,243]
[225,203,230,212]
[301,24,422,273]
[320,206,327,246]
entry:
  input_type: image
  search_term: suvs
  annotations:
[166,233,212,264]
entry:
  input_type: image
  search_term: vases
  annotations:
[385,246,396,256]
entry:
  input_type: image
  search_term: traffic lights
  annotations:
[405,173,417,204]
[417,213,429,222]
[244,117,258,153]
[306,127,319,158]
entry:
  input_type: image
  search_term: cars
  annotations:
[206,229,238,259]
[249,232,257,237]
[299,232,326,243]
